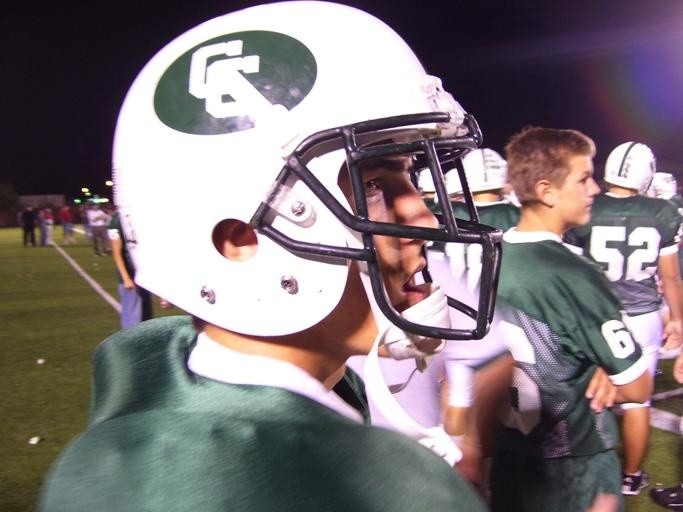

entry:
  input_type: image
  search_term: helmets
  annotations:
[645,172,676,200]
[604,141,656,194]
[433,168,464,204]
[460,147,507,193]
[416,167,435,193]
[111,1,504,340]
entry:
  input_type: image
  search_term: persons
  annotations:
[347,125,683,512]
[32,0,506,512]
[16,198,174,331]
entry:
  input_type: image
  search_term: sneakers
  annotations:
[619,469,649,496]
[649,483,682,512]
